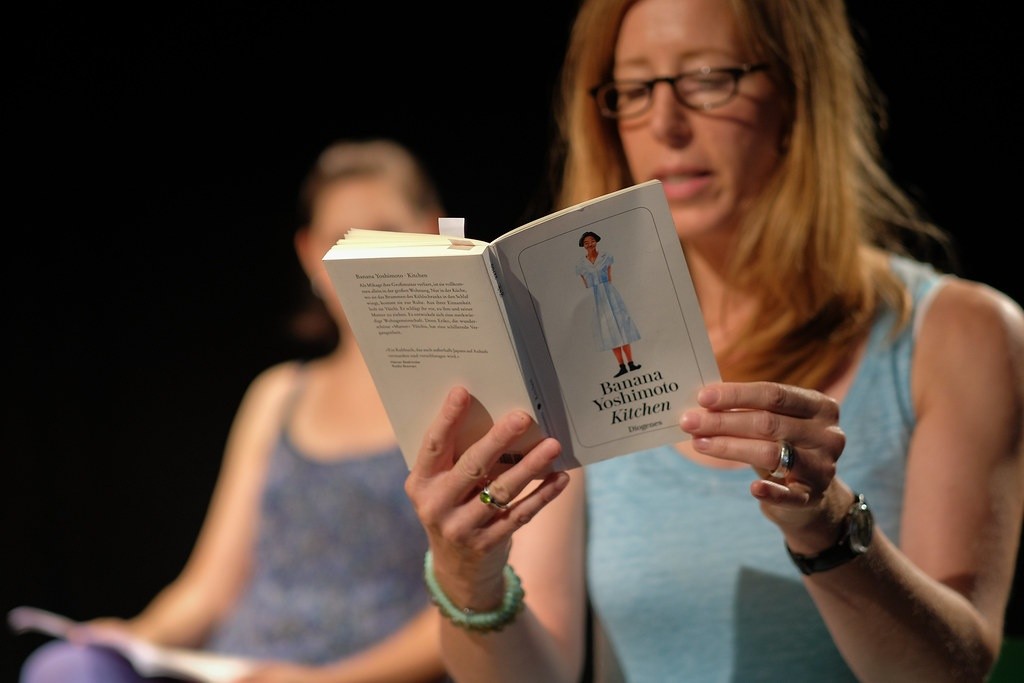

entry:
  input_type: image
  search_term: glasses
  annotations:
[587,59,778,118]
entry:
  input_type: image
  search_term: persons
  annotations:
[575,231,642,379]
[403,0,1024,683]
[15,142,444,683]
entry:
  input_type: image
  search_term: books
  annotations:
[9,604,260,682]
[323,179,723,479]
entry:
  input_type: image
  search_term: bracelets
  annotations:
[425,547,525,632]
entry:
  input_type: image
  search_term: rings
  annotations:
[479,482,509,512]
[766,439,796,480]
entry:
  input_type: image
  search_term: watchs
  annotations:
[782,490,875,576]
